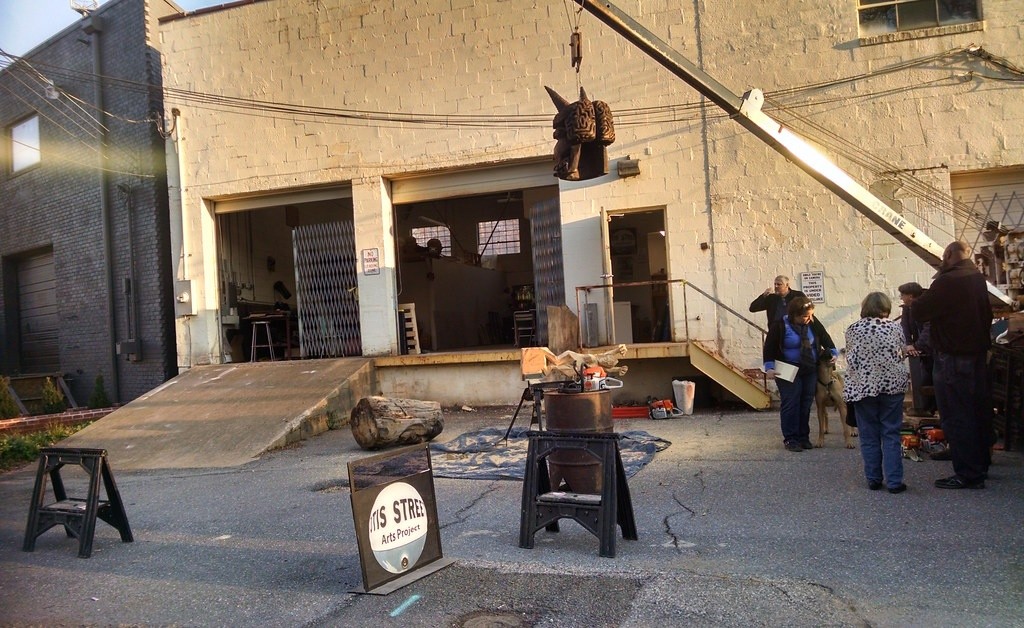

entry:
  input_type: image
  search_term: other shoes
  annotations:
[886,483,907,494]
[785,441,813,452]
[868,481,883,490]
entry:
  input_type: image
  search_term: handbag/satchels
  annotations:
[845,400,861,428]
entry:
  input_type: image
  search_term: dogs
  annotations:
[815,345,858,449]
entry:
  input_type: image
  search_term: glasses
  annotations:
[899,294,904,298]
[801,301,813,308]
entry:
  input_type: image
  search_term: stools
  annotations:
[249,322,275,362]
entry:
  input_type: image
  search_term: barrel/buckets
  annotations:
[544,390,614,493]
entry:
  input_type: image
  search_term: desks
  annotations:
[243,315,298,360]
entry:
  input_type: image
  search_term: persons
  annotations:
[843,292,908,493]
[909,242,994,490]
[763,296,837,452]
[749,276,807,330]
[898,283,936,417]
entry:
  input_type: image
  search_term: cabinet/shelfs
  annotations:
[514,308,536,348]
[613,301,633,345]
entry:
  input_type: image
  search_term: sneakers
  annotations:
[934,467,990,490]
